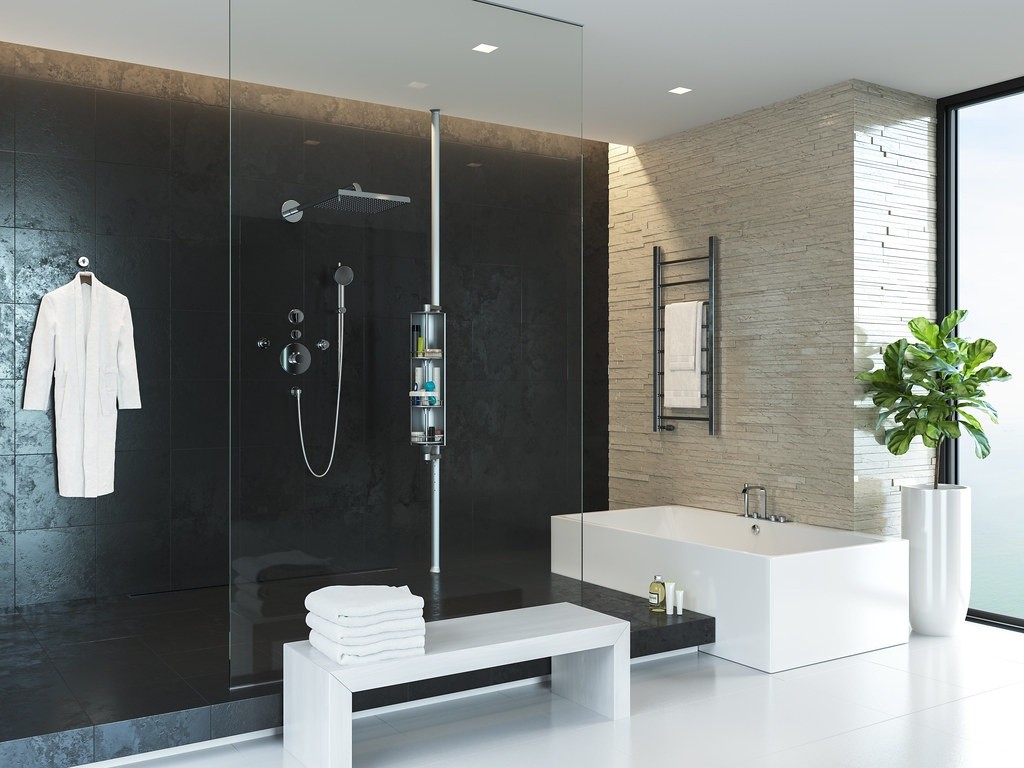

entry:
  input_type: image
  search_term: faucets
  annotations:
[741,485,767,521]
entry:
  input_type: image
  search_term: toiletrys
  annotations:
[410,323,445,446]
[648,574,685,617]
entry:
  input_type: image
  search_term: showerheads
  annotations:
[335,265,354,380]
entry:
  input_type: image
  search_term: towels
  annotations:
[663,300,709,410]
[305,584,427,667]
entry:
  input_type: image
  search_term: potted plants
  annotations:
[855,310,1012,637]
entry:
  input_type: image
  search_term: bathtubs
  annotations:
[550,504,911,675]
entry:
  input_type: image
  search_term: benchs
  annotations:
[284,602,632,767]
